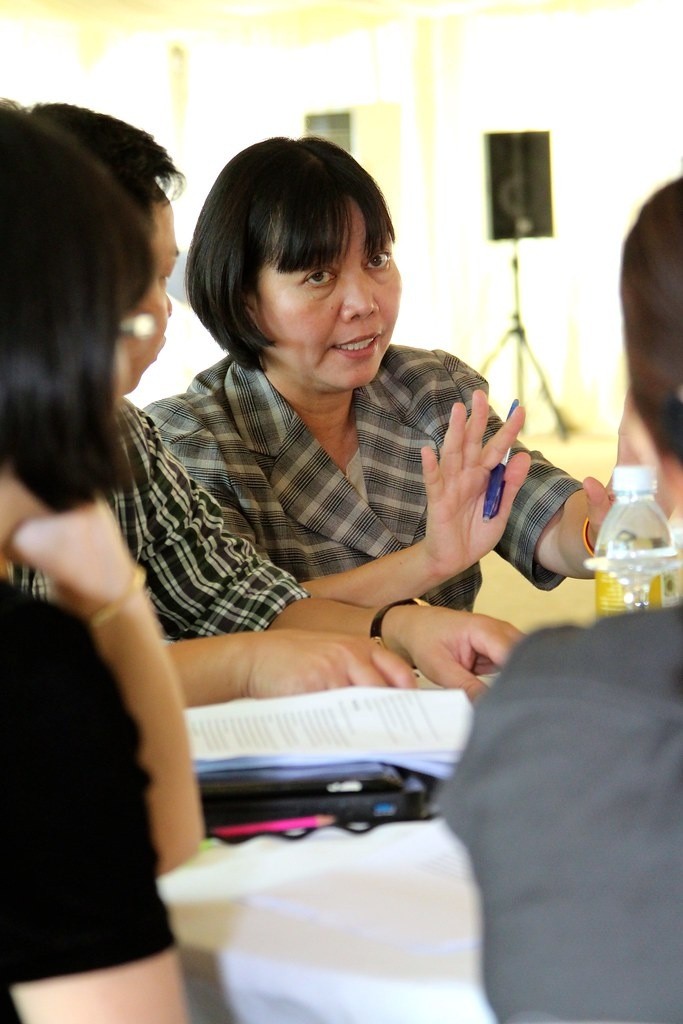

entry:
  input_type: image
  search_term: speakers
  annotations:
[484,131,554,240]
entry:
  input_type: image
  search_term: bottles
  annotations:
[586,464,679,621]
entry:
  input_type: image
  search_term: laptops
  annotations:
[196,758,427,823]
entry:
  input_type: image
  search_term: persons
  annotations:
[24,104,530,707]
[0,97,208,985]
[139,132,678,615]
[438,178,683,1024]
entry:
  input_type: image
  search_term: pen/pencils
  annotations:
[209,814,339,840]
[480,398,521,525]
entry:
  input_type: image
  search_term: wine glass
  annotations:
[582,546,678,613]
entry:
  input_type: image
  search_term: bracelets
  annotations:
[87,566,151,632]
[368,597,430,678]
[582,514,595,558]
